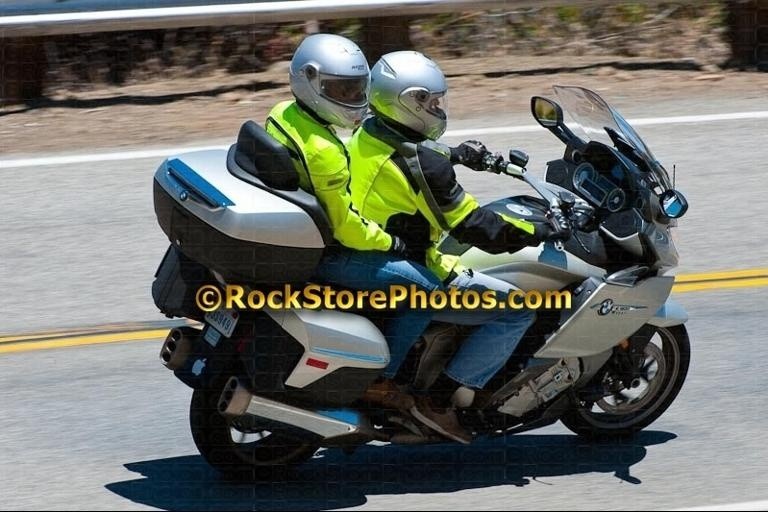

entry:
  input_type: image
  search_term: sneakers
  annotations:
[365,377,416,412]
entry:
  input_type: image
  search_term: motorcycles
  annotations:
[145,84,690,484]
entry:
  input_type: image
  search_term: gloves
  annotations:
[449,140,487,171]
[538,215,571,243]
[394,233,426,262]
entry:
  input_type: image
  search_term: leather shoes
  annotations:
[410,395,472,445]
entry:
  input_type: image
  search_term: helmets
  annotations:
[367,50,450,144]
[288,33,371,131]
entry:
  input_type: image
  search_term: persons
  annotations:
[262,30,447,413]
[343,43,572,447]
